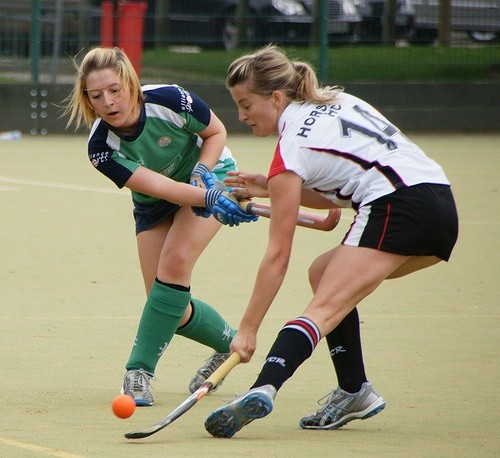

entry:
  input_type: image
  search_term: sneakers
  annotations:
[299,379,387,430]
[119,368,158,406]
[203,384,277,438]
[188,350,232,397]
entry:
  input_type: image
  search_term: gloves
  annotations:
[189,162,216,218]
[205,188,259,227]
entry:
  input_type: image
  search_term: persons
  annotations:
[57,46,259,406]
[205,45,459,439]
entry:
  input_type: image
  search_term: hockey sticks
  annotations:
[239,193,343,231]
[124,350,242,440]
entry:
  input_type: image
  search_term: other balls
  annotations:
[112,394,136,420]
